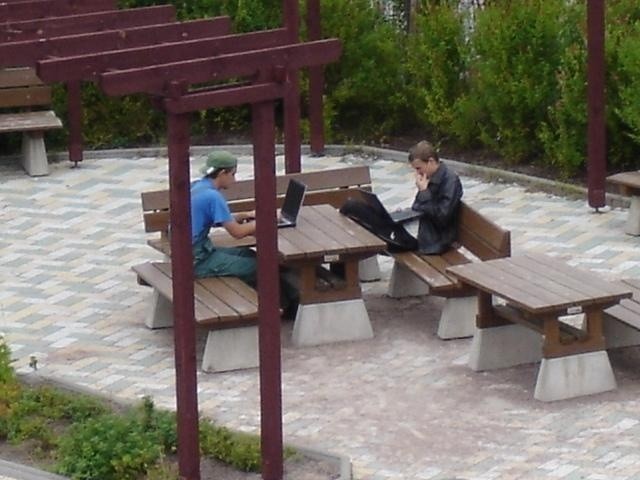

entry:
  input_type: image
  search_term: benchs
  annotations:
[0,67,64,178]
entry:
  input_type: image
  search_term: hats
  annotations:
[198,151,237,175]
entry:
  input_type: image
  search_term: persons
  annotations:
[330,140,463,278]
[169,151,299,320]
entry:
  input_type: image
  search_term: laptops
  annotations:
[245,177,308,228]
[356,188,424,226]
